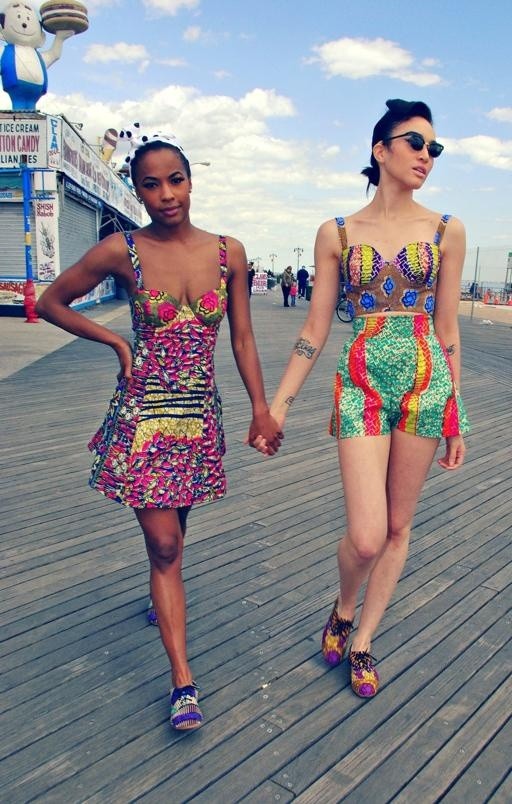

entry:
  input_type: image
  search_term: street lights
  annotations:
[248,253,277,275]
[293,246,305,276]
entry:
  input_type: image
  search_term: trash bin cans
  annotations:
[306,286,312,301]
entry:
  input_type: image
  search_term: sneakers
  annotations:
[348,644,379,699]
[147,597,160,625]
[321,594,355,667]
[169,680,204,730]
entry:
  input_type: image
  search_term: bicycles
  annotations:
[337,296,352,323]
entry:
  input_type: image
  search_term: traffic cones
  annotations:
[506,292,512,306]
[493,291,500,305]
[483,289,490,304]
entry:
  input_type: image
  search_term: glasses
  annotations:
[387,131,446,160]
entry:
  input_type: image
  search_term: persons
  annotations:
[248,262,255,299]
[280,266,296,307]
[243,99,473,697]
[32,140,284,730]
[297,266,309,297]
[469,283,483,300]
[289,282,299,307]
